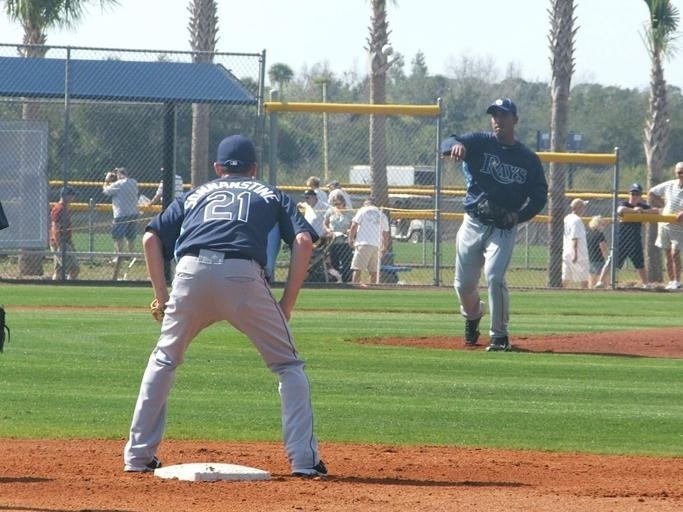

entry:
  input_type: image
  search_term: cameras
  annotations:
[104,168,118,179]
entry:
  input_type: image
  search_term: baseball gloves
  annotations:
[476,199,514,229]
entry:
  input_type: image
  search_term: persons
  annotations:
[441,98,548,351]
[587,216,610,288]
[295,176,390,285]
[647,162,683,289]
[147,167,183,211]
[49,187,80,281]
[124,134,328,476]
[102,167,139,264]
[595,183,659,288]
[562,198,590,288]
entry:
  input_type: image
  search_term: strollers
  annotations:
[293,232,342,284]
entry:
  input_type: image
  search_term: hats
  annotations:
[487,97,518,115]
[570,197,589,211]
[628,182,643,192]
[300,189,316,197]
[216,133,259,166]
[60,186,80,196]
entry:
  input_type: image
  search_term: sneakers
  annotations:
[464,317,479,345]
[666,281,680,290]
[487,332,509,352]
[642,284,652,289]
[593,281,604,290]
[124,454,161,472]
[291,460,328,477]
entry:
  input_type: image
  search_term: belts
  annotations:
[180,247,254,260]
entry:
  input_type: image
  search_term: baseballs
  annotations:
[381,44,394,56]
